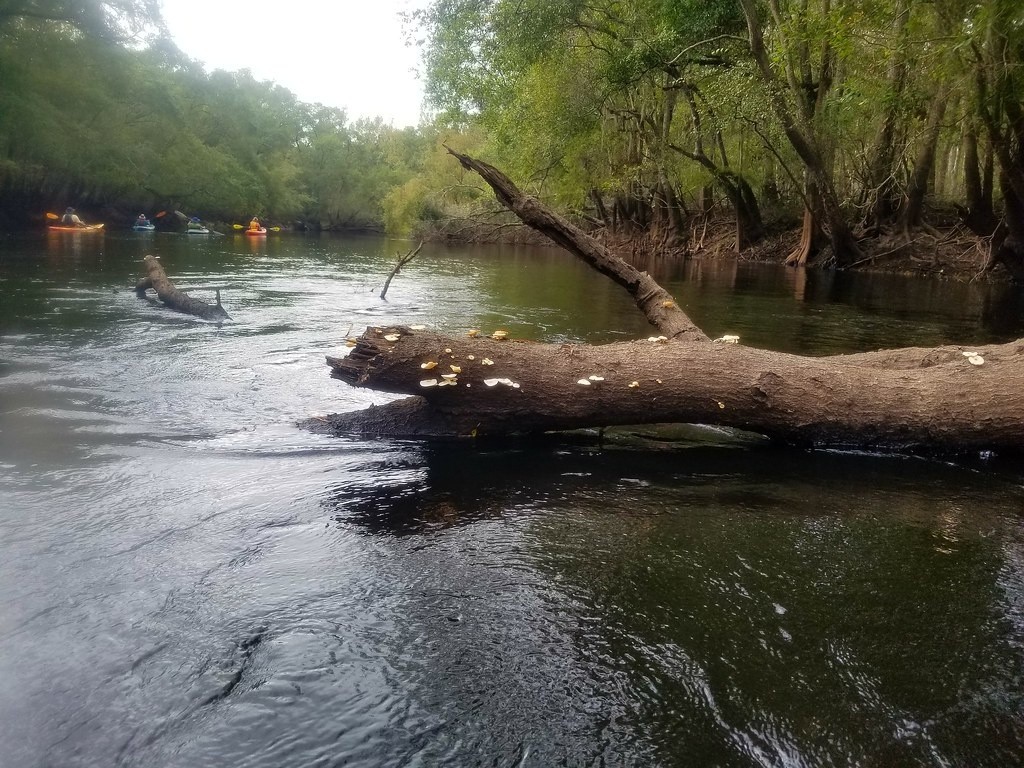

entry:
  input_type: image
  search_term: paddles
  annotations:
[174,210,197,224]
[149,211,165,219]
[233,224,279,232]
[47,212,94,229]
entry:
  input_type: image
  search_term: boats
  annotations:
[245,226,267,235]
[134,224,156,231]
[48,223,105,232]
[187,227,210,234]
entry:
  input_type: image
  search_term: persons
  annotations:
[138,214,147,225]
[249,216,262,231]
[61,207,85,228]
[190,216,202,223]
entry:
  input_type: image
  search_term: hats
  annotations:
[66,207,75,214]
[253,217,258,221]
[191,217,200,222]
[139,214,146,219]
